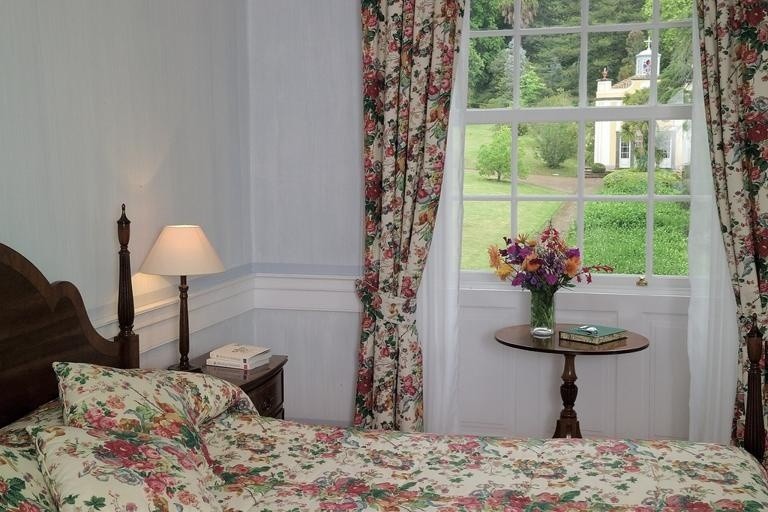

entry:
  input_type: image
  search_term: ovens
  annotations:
[0,243,768,512]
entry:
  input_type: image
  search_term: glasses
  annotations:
[139,224,225,374]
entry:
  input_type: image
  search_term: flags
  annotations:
[528,293,556,338]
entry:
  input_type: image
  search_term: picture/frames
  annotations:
[494,324,650,438]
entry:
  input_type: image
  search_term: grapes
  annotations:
[168,351,289,417]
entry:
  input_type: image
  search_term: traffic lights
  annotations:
[27,357,259,511]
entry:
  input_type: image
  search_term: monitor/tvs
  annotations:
[209,342,272,362]
[206,357,270,371]
[558,324,628,346]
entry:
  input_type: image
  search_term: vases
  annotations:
[488,223,614,331]
